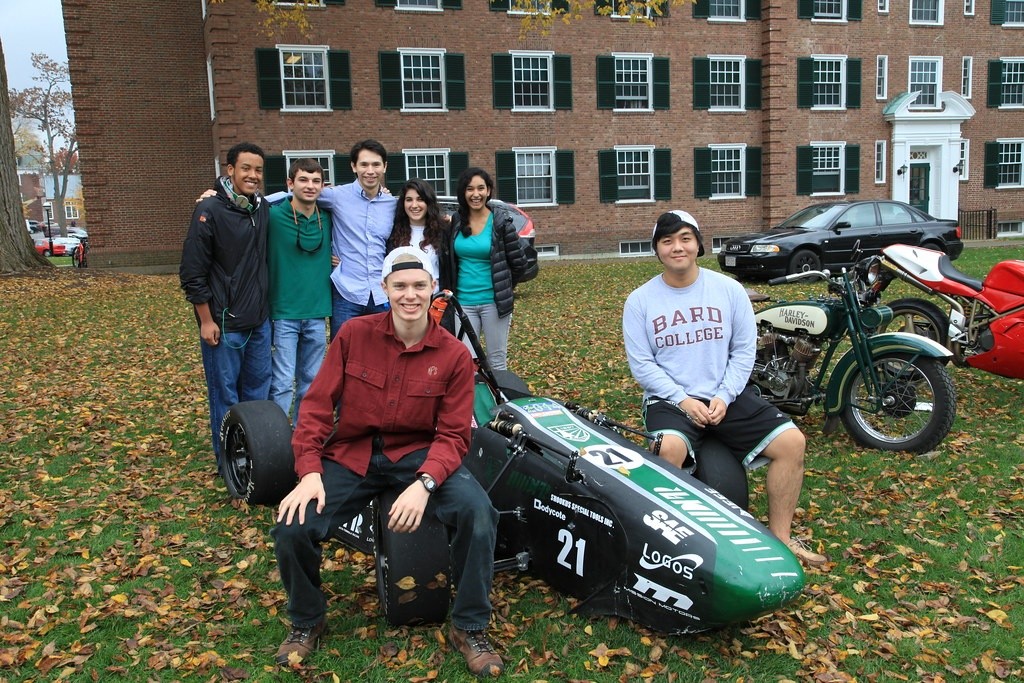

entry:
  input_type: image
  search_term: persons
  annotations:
[332,177,452,337]
[263,140,454,427]
[179,142,274,474]
[622,210,828,564]
[194,157,392,435]
[452,167,527,374]
[271,245,501,677]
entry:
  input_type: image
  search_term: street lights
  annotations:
[43,202,54,257]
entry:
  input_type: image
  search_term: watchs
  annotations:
[418,476,436,495]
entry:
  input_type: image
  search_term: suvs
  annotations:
[392,195,535,251]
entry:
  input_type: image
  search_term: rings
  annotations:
[694,417,699,421]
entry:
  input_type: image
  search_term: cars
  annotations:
[26,220,87,255]
[718,199,963,283]
[34,239,66,256]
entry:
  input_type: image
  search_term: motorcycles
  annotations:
[744,238,956,457]
[872,243,1024,382]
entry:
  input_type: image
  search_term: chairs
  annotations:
[465,379,499,431]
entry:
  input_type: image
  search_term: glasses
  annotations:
[296,224,324,253]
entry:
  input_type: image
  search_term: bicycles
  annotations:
[72,235,87,267]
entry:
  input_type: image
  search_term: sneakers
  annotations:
[447,625,505,677]
[787,536,828,568]
[276,621,328,668]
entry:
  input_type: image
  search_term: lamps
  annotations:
[898,164,908,181]
[953,160,964,179]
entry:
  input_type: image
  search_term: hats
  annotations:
[652,210,705,263]
[381,246,436,282]
